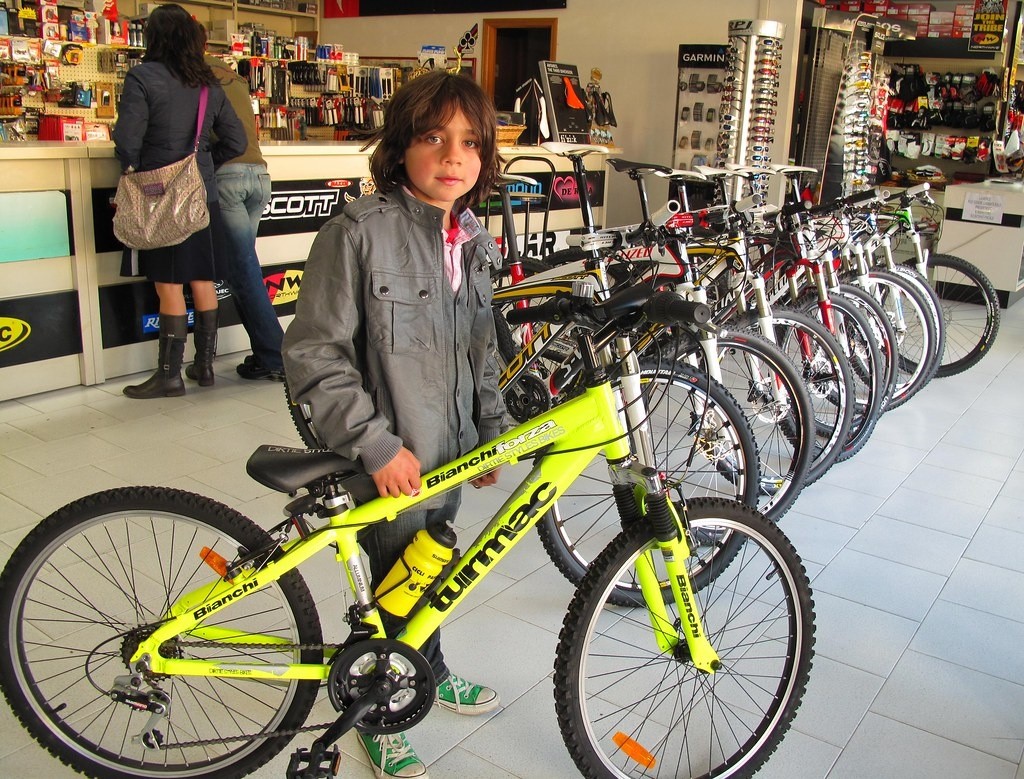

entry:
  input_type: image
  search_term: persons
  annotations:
[199,24,285,381]
[113,4,248,399]
[280,73,508,778]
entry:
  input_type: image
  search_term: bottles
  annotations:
[375,520,458,618]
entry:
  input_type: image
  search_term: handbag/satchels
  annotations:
[112,153,210,250]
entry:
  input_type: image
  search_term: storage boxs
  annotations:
[816,0,976,38]
[0,2,393,140]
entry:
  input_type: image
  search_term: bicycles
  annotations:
[276,139,1000,606]
[0,248,816,779]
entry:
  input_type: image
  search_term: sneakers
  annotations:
[237,356,286,381]
[433,674,500,716]
[357,729,430,779]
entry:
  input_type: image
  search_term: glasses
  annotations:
[712,37,747,206]
[841,51,871,193]
[743,38,783,208]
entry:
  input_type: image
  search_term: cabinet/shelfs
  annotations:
[875,38,1013,182]
[0,0,320,141]
[717,19,787,204]
[801,24,888,200]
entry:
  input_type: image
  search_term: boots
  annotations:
[123,313,188,398]
[185,306,219,386]
